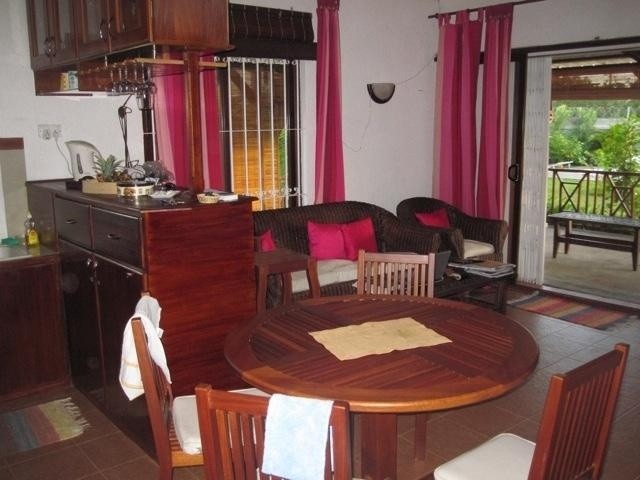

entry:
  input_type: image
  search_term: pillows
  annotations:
[339,215,378,261]
[414,208,450,229]
[307,219,347,262]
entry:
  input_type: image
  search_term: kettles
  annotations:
[65,140,103,180]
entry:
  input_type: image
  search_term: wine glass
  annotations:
[106,66,157,109]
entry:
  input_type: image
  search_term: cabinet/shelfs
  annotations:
[25,0,235,96]
[25,178,258,467]
[0,237,75,415]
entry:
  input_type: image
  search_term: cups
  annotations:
[68,71,78,91]
[60,73,69,92]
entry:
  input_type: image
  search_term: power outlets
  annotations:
[38,124,66,139]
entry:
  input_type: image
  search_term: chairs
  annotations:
[397,197,510,264]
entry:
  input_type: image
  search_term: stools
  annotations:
[253,249,322,312]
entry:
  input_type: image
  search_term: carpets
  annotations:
[506,290,640,336]
[0,396,91,458]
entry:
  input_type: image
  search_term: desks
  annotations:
[547,211,640,271]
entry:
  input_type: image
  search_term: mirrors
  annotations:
[0,138,29,243]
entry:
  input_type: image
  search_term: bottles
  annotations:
[23,212,40,247]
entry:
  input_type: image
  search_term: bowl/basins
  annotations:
[197,193,219,203]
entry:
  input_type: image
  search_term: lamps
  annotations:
[367,83,396,104]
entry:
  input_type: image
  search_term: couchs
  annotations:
[258,229,276,253]
[253,201,442,306]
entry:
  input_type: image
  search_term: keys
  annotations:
[160,198,185,207]
